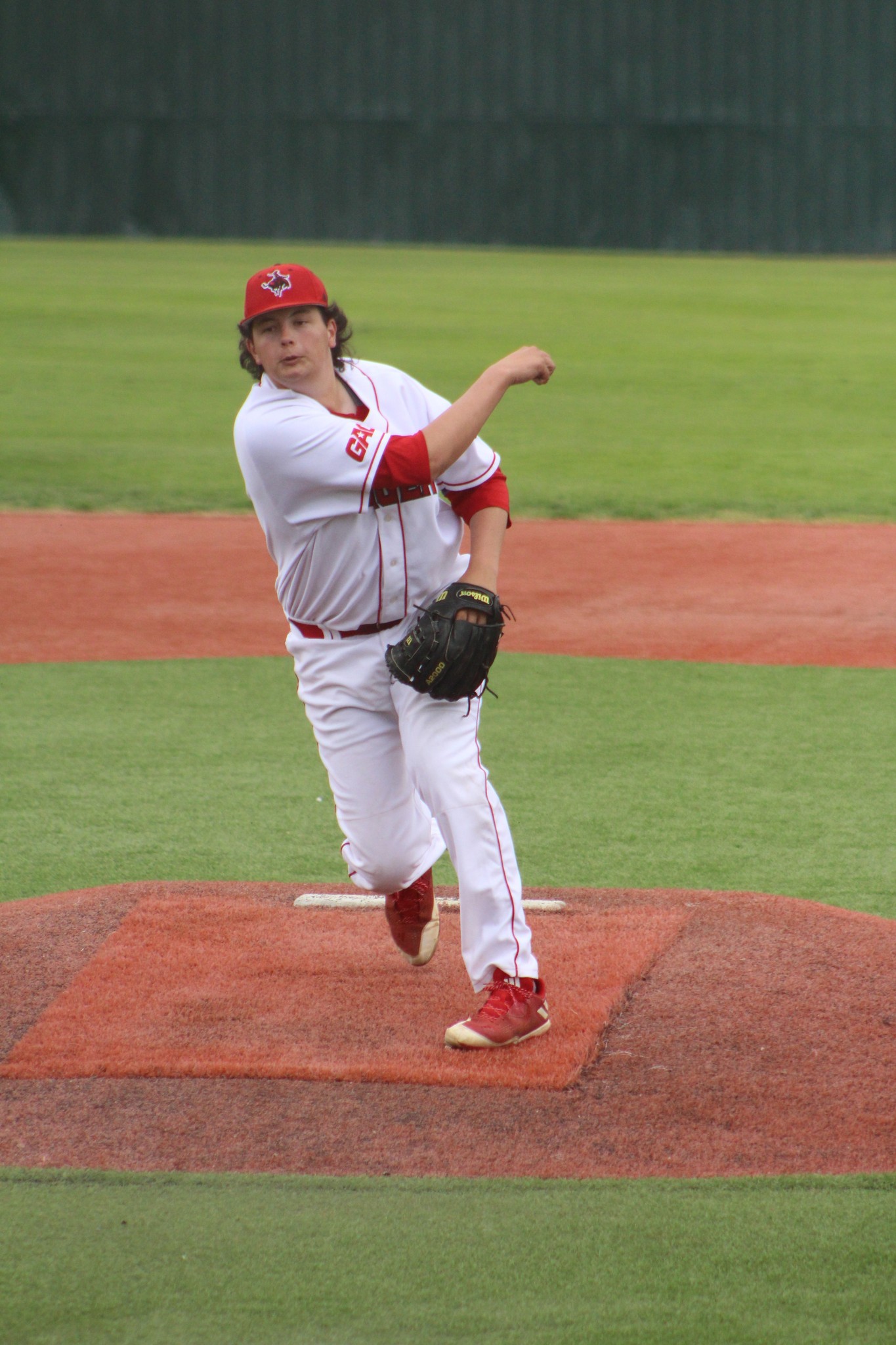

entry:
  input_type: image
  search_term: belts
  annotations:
[289,618,402,639]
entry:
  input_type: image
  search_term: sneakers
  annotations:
[443,969,551,1047]
[384,866,440,965]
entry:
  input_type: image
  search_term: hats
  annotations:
[240,264,327,325]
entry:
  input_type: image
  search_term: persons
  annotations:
[232,262,556,1050]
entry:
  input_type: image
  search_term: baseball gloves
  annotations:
[384,582,505,703]
